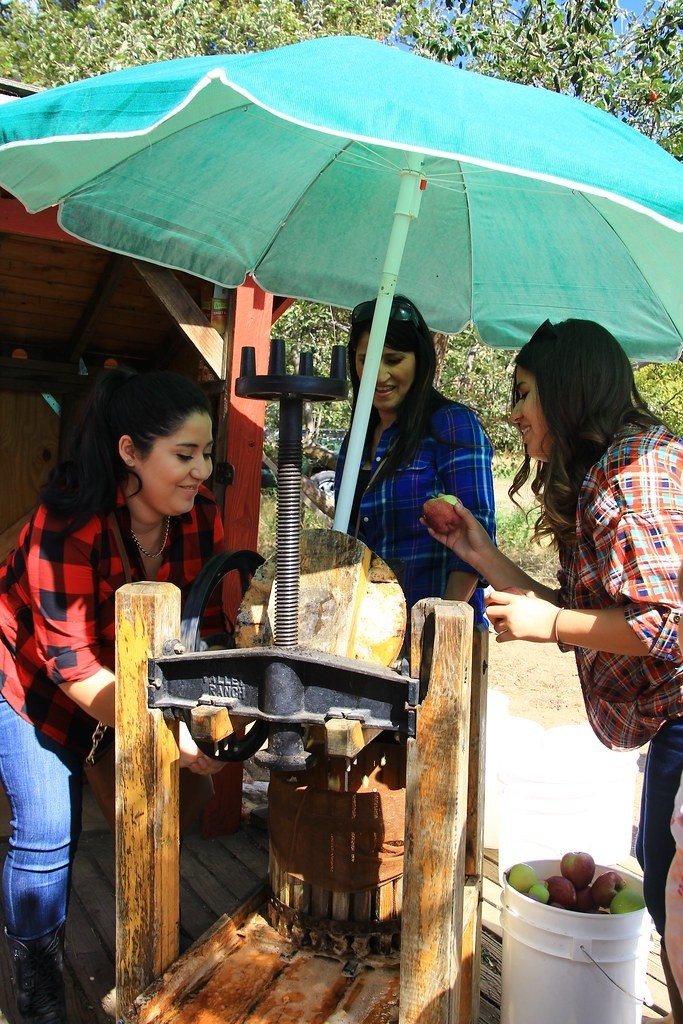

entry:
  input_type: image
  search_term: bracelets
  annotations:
[555,605,570,644]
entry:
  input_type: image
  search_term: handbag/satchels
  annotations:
[67,720,115,768]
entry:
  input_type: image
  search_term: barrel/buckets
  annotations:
[498,861,655,1023]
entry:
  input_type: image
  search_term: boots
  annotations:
[4,918,100,1024]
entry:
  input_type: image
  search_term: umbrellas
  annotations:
[0,35,683,535]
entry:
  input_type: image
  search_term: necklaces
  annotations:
[131,516,170,559]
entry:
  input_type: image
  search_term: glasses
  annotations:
[352,300,419,328]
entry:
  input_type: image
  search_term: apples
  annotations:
[422,495,464,534]
[504,851,645,914]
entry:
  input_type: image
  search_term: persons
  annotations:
[335,296,497,688]
[420,318,683,1024]
[0,364,231,1024]
